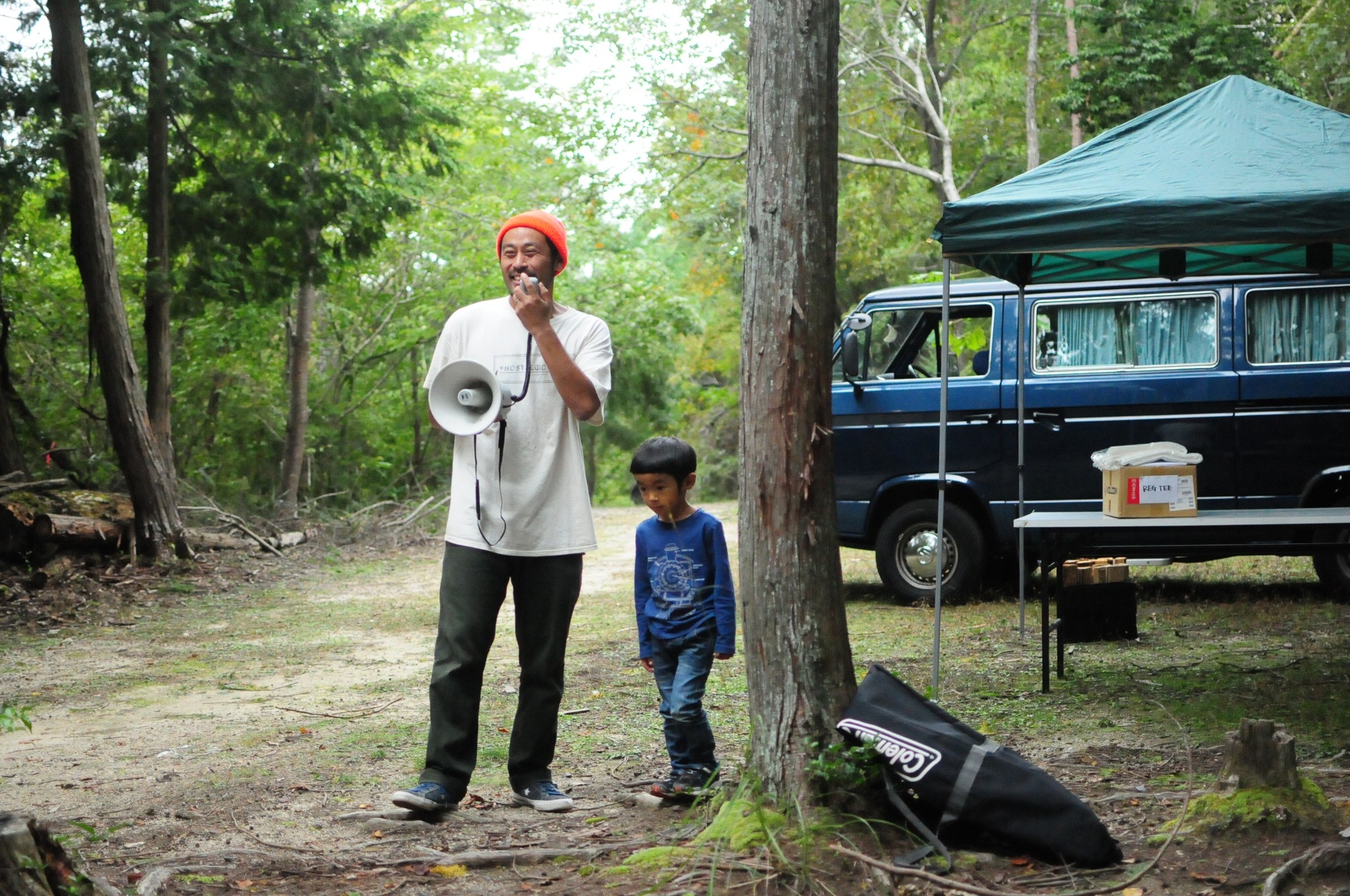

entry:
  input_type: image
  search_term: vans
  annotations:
[830,267,1349,609]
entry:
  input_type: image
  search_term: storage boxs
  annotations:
[1059,581,1139,645]
[1102,464,1198,518]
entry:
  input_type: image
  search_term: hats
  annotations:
[495,210,568,278]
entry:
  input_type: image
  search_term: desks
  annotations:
[1013,508,1350,694]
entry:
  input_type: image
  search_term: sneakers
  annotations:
[649,767,687,799]
[673,764,723,797]
[392,781,460,812]
[512,781,573,811]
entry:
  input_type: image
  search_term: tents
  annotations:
[931,75,1350,706]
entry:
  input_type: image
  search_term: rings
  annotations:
[515,298,519,304]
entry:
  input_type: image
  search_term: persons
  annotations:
[630,435,735,803]
[389,210,614,815]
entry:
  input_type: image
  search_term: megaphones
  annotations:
[429,358,514,436]
